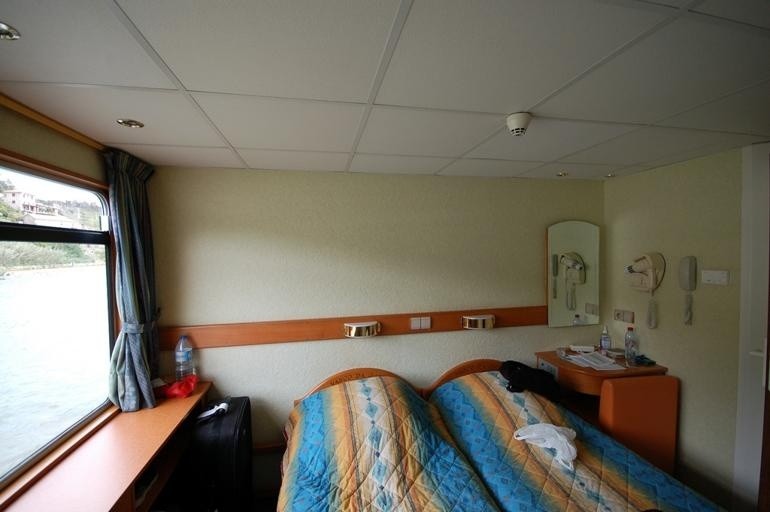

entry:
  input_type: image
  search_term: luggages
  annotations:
[175,397,252,512]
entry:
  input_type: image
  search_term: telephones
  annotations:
[677,256,697,291]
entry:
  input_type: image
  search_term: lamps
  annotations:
[462,314,497,331]
[344,321,382,339]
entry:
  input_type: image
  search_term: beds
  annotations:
[275,358,729,512]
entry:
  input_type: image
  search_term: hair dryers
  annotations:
[626,259,658,290]
[559,254,584,280]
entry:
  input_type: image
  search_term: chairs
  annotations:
[568,376,679,475]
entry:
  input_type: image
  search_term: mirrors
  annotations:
[546,218,602,328]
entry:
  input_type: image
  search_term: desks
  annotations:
[535,346,669,396]
[3,381,214,511]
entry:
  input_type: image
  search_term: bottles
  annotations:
[624,327,635,360]
[174,334,194,384]
[572,314,584,324]
[600,325,611,349]
